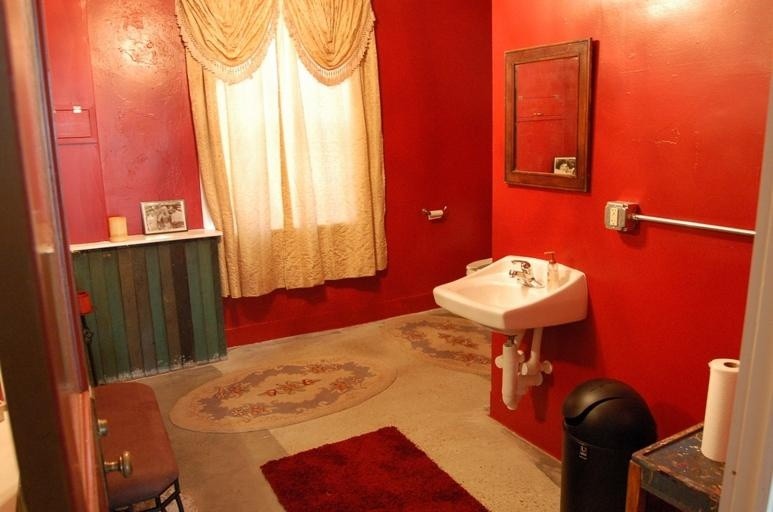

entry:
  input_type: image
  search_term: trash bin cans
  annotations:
[561,378,656,511]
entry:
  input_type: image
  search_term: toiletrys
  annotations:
[544,250,561,292]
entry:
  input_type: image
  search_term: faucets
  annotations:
[507,259,543,291]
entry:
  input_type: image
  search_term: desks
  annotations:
[69,226,228,388]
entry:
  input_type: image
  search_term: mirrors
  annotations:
[502,37,595,192]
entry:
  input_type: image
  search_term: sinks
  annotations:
[432,254,588,339]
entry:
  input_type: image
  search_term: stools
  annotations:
[93,382,185,512]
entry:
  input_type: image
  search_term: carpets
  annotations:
[260,426,491,512]
[381,313,491,376]
[168,355,398,434]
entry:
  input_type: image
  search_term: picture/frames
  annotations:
[554,157,576,176]
[139,198,188,235]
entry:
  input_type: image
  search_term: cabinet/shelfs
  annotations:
[625,421,725,511]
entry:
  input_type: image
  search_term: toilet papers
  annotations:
[428,209,445,222]
[699,357,741,465]
